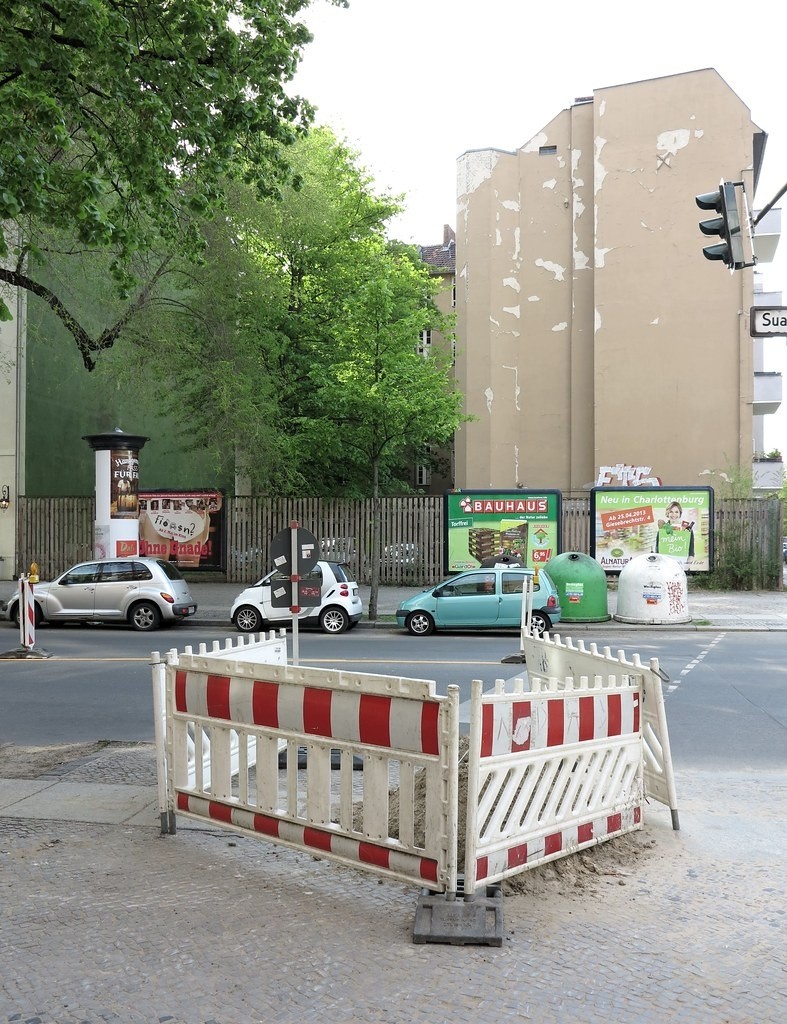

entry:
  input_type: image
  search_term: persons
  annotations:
[655,501,694,563]
[117,476,130,495]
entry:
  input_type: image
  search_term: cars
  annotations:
[5,556,197,631]
[396,568,561,638]
[229,560,364,634]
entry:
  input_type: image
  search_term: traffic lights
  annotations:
[695,182,746,270]
